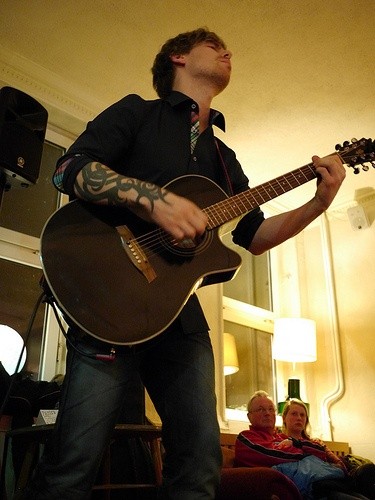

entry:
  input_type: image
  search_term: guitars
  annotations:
[40,137,375,354]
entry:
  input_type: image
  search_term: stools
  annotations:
[6,424,166,500]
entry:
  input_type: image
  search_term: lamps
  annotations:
[223,333,239,376]
[272,317,318,413]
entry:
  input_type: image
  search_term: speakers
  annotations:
[117,382,145,425]
[347,187,375,229]
[0,85,48,184]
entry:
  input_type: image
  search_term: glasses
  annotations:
[250,407,277,414]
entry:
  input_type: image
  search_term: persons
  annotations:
[31,27,346,500]
[235,389,374,500]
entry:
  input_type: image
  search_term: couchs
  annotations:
[219,433,302,500]
